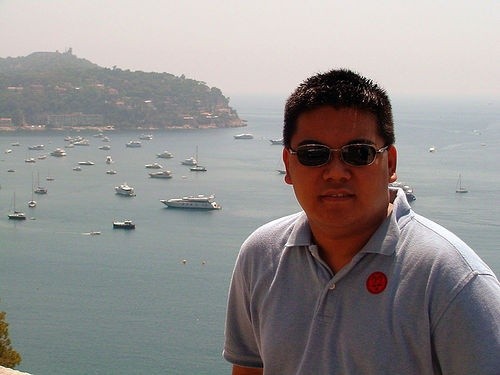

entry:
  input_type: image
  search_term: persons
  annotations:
[223,68,500,375]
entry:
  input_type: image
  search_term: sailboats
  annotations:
[27,171,37,207]
[456,170,470,193]
[34,170,48,194]
[7,192,26,219]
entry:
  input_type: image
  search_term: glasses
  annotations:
[289,143,389,168]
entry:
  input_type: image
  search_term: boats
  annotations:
[37,155,47,159]
[139,134,153,139]
[28,144,46,150]
[12,142,20,146]
[106,169,117,174]
[160,194,222,210]
[183,157,198,165]
[156,150,174,158]
[105,156,113,164]
[145,161,164,170]
[63,135,90,147]
[389,183,417,201]
[148,170,172,178]
[113,219,136,229]
[190,165,208,171]
[125,139,142,147]
[98,145,111,150]
[72,166,82,171]
[25,157,37,162]
[115,184,134,195]
[89,230,101,235]
[100,136,111,142]
[5,148,13,154]
[269,137,283,145]
[233,133,254,139]
[50,147,67,156]
[93,133,105,138]
[78,161,95,165]
[8,169,16,172]
